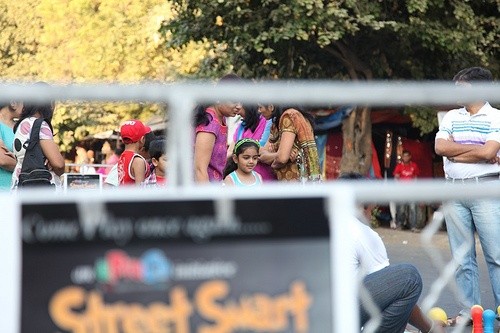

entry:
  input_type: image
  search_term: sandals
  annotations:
[447,314,472,326]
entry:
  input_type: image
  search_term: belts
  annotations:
[446,175,499,182]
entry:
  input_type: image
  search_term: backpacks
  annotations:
[18,118,55,191]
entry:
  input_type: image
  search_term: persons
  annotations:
[340,171,423,333]
[10,81,65,190]
[194,74,243,183]
[435,67,500,326]
[75,141,116,174]
[0,84,23,190]
[257,100,320,181]
[118,120,151,188]
[361,138,382,220]
[394,150,418,229]
[224,140,265,185]
[144,142,176,185]
[236,100,277,181]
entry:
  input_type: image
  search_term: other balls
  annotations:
[428,307,447,323]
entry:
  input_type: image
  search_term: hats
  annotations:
[121,120,151,144]
